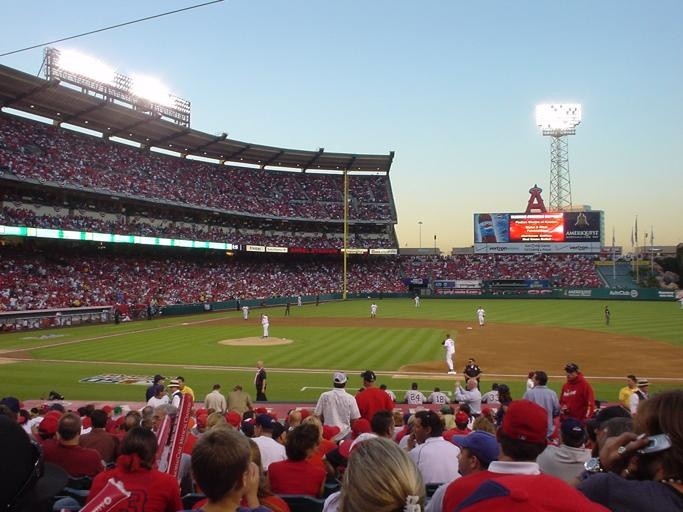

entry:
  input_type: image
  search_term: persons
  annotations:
[605,306,610,325]
[399,254,606,294]
[415,295,420,307]
[189,427,274,511]
[314,370,509,432]
[523,364,593,447]
[0,397,40,510]
[619,375,648,416]
[265,425,327,499]
[424,430,499,511]
[1,114,393,247]
[321,435,423,512]
[16,403,646,471]
[252,363,272,402]
[477,306,486,327]
[461,357,482,390]
[1,241,408,322]
[572,390,683,511]
[262,314,269,337]
[86,427,181,512]
[440,333,459,377]
[192,438,289,510]
[147,375,254,414]
[442,401,611,511]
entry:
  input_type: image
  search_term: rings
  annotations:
[624,468,632,477]
[617,445,627,456]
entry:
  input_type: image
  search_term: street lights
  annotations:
[434,234,437,253]
[416,219,425,248]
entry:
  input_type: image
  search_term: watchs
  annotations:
[582,457,601,473]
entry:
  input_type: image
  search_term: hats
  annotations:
[564,364,577,371]
[155,375,165,380]
[360,371,376,382]
[439,400,584,462]
[636,380,650,386]
[333,372,348,384]
[167,380,180,387]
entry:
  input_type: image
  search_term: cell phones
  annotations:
[632,434,674,455]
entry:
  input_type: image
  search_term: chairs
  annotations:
[276,494,327,511]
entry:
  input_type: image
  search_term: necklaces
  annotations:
[656,475,681,485]
